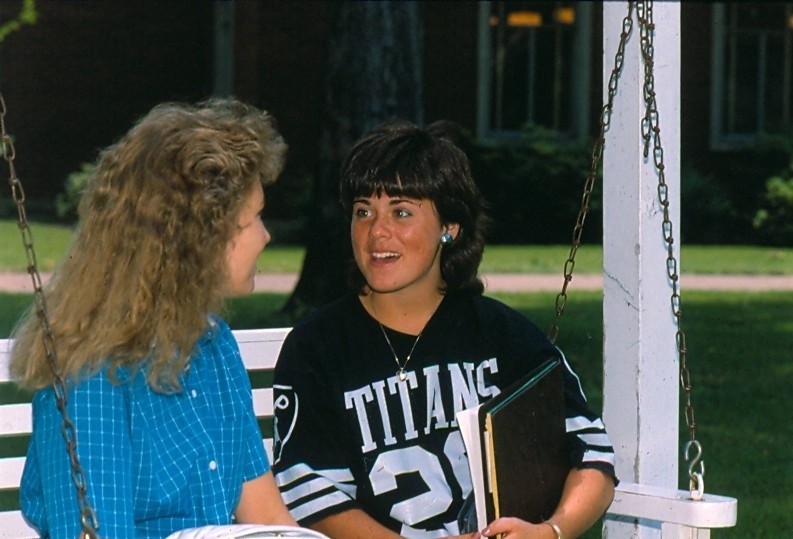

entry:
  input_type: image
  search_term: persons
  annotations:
[272,120,620,539]
[8,97,298,539]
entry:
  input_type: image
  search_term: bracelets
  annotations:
[545,520,564,539]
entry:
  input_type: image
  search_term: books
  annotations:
[455,357,570,539]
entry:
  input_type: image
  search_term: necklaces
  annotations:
[380,322,422,382]
